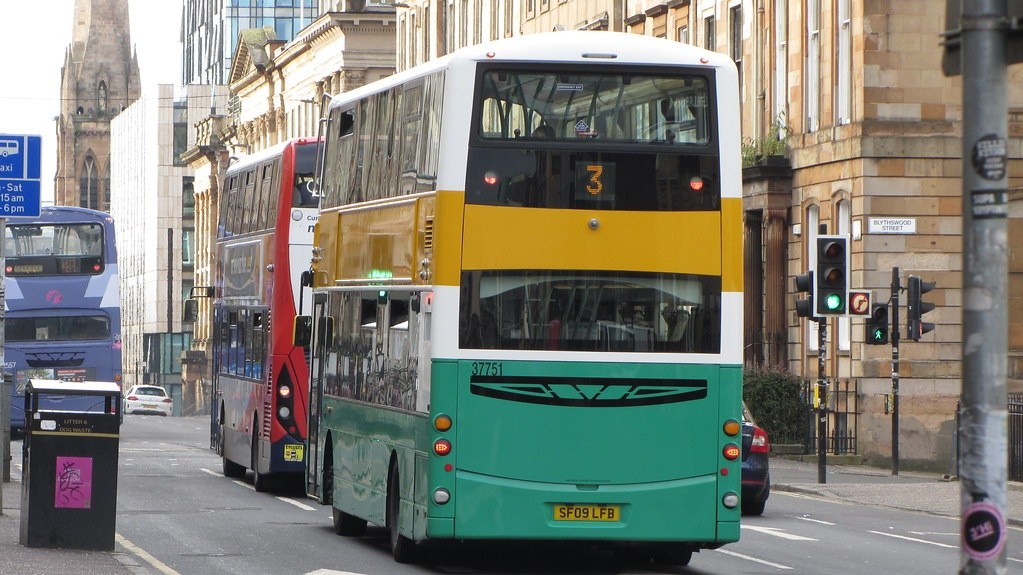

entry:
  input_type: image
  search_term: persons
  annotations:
[527,124,571,182]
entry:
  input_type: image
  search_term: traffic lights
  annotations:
[865,302,890,345]
[812,234,852,317]
[907,275,937,343]
[796,270,819,323]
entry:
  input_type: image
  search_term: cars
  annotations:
[124,385,173,417]
[742,401,771,516]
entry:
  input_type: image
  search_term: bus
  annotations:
[181,134,417,492]
[5,205,124,437]
[290,29,745,566]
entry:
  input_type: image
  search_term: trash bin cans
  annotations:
[19,378,124,551]
